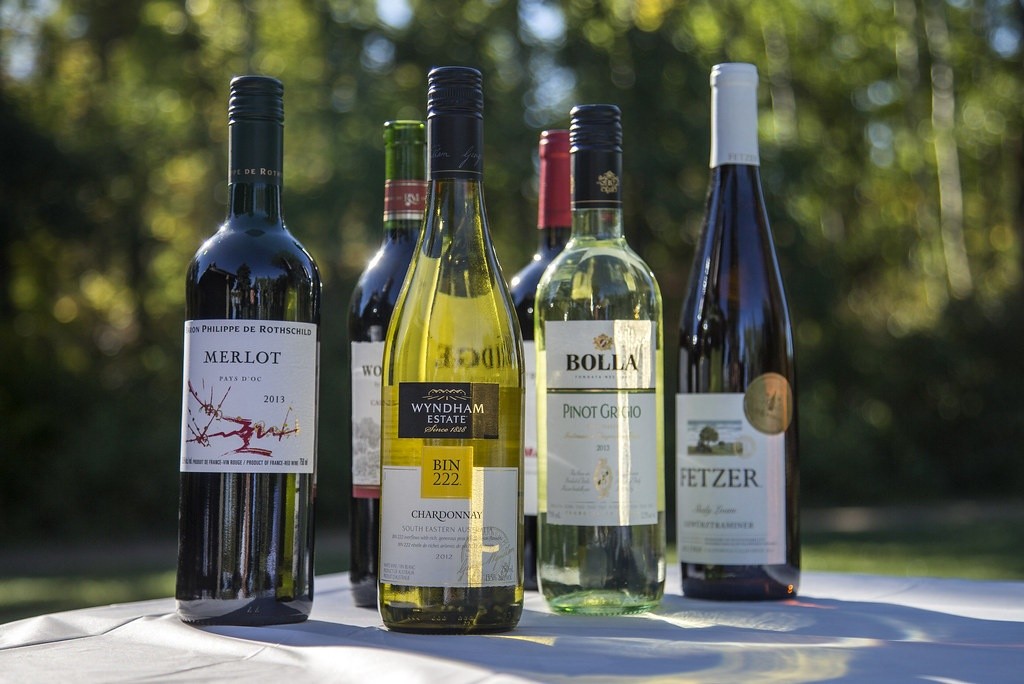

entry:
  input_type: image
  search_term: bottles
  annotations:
[521,127,571,589]
[377,66,527,635]
[676,63,798,600]
[175,76,326,627]
[535,102,669,619]
[347,118,431,607]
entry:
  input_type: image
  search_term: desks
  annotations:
[1,566,1024,684]
